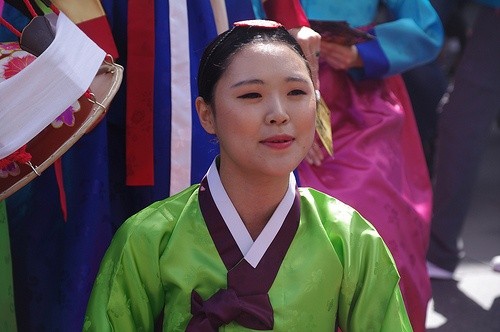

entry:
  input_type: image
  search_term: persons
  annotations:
[424,0,500,280]
[81,19,414,332]
[263,0,444,332]
